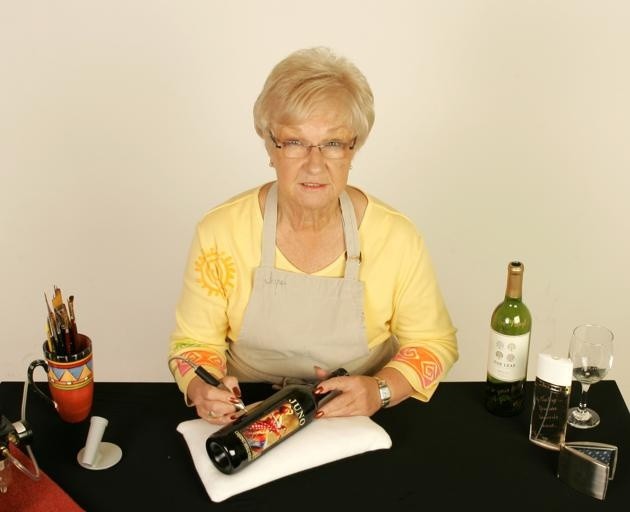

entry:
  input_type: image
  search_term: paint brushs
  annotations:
[44,285,80,355]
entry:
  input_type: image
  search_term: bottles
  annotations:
[206,368,348,475]
[485,261,532,417]
[528,354,573,451]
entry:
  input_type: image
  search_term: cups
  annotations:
[27,334,94,423]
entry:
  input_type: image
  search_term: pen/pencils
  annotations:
[186,360,248,414]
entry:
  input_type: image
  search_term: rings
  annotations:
[207,408,217,420]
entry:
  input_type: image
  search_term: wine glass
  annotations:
[568,324,614,431]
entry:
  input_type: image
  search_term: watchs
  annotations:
[372,374,391,410]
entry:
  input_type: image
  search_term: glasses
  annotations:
[269,131,357,159]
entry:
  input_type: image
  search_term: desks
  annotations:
[2,382,629,512]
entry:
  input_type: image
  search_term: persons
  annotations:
[168,42,460,425]
